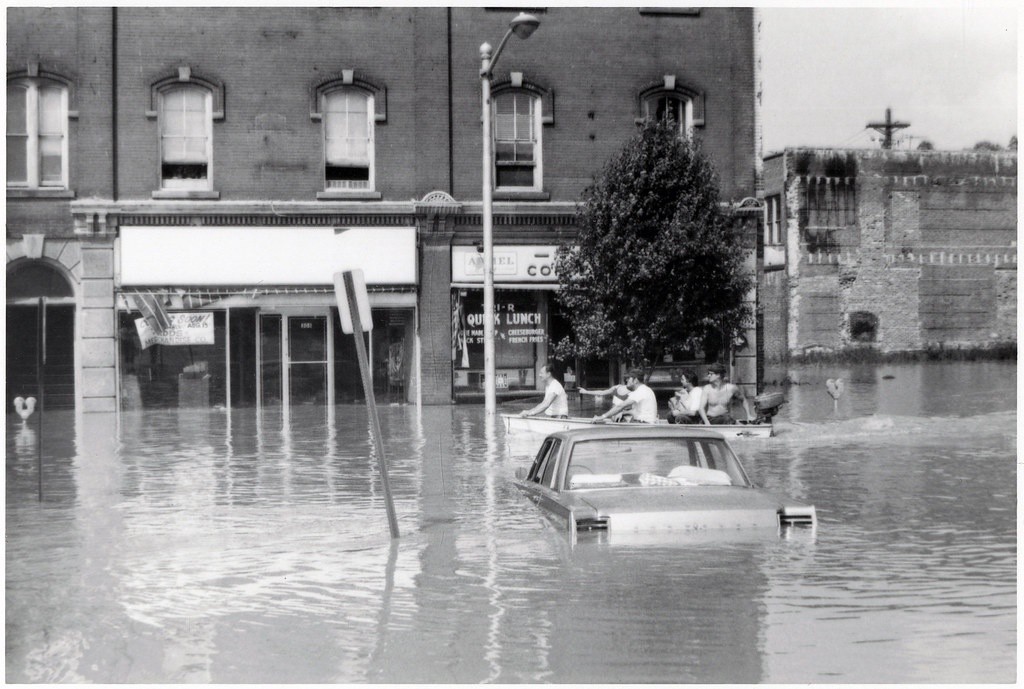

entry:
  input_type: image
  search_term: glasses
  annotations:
[708,371,717,375]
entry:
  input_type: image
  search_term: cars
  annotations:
[516,420,820,553]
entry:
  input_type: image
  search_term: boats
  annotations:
[498,387,791,454]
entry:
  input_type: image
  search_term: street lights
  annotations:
[479,11,546,431]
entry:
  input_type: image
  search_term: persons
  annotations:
[519,366,570,419]
[576,362,753,426]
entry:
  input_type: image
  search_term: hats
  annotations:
[624,369,644,378]
[708,363,727,373]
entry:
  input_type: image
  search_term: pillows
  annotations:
[667,466,732,486]
